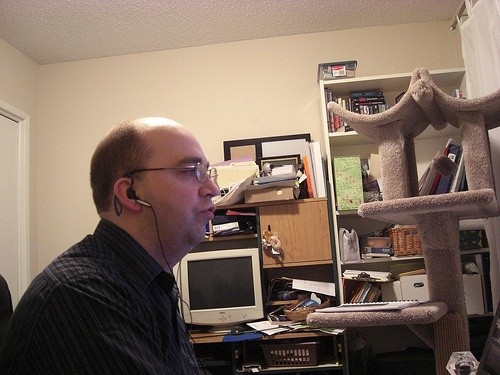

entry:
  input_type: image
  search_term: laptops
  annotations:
[476,303,500,375]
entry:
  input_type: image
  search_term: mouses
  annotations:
[232,326,244,335]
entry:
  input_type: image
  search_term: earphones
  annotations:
[127,189,150,207]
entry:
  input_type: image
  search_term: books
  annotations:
[325,88,394,133]
[315,300,420,312]
[342,279,380,304]
[302,139,327,198]
[450,89,462,98]
[419,141,465,196]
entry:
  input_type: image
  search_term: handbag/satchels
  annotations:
[339,227,361,263]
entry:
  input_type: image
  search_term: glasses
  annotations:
[125,161,218,183]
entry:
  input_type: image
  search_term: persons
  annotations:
[0,117,220,375]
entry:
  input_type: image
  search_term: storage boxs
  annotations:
[318,60,357,81]
[381,273,484,316]
[244,180,295,203]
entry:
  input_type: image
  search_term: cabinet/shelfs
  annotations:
[188,197,347,375]
[306,68,500,375]
[319,67,500,319]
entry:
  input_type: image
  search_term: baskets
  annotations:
[259,340,323,367]
[386,226,424,257]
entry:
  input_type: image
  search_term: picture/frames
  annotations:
[259,154,301,177]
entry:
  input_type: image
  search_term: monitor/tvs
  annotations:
[180,247,264,334]
